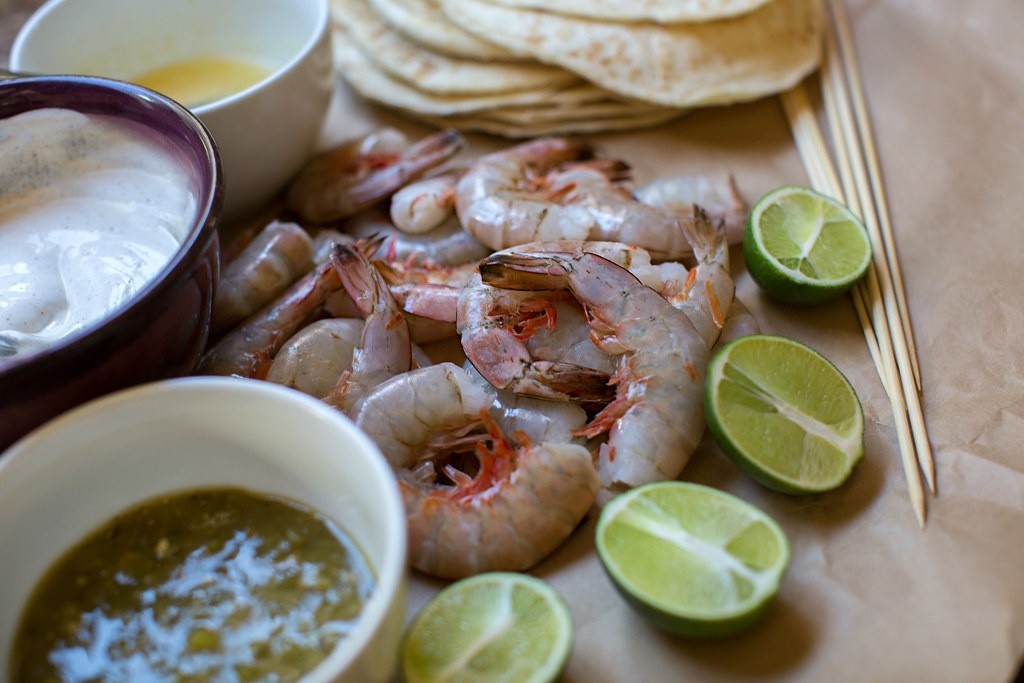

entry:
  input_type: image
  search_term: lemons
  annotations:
[595,480,790,642]
[704,335,864,495]
[743,185,873,304]
[398,573,576,683]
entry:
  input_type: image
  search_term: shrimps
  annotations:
[189,125,751,582]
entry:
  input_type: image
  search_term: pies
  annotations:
[327,1,827,137]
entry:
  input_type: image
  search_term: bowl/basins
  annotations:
[0,75,219,456]
[5,0,336,214]
[0,379,407,683]
